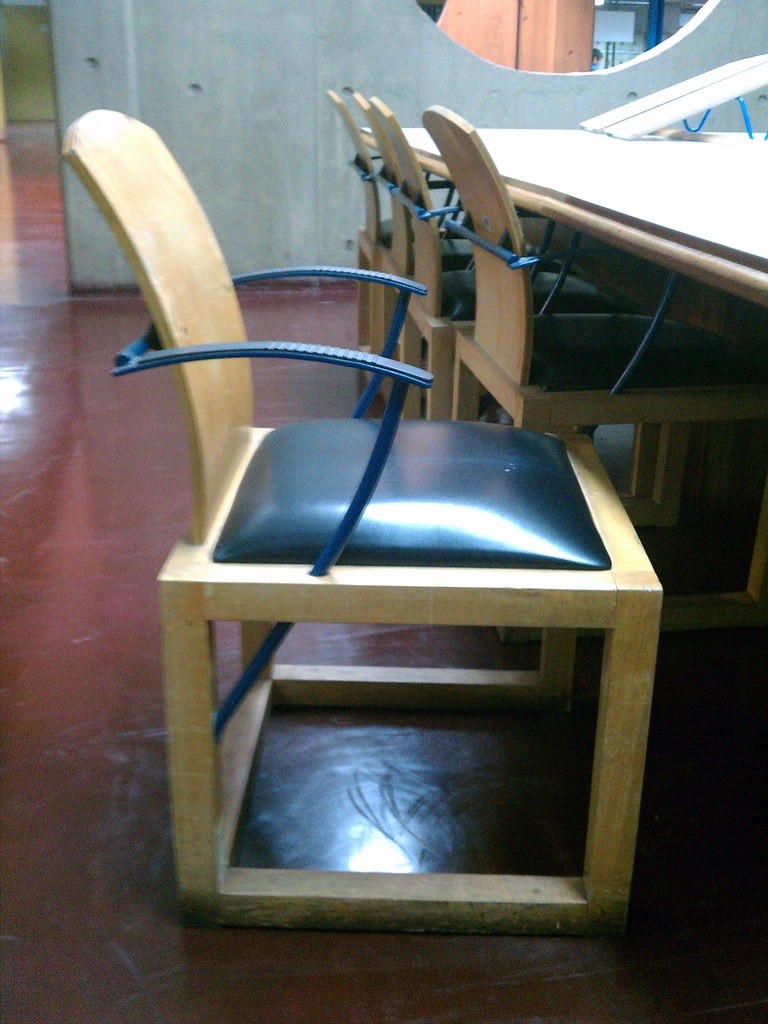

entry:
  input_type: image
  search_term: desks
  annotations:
[360,127,768,306]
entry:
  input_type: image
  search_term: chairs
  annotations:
[327,84,768,645]
[61,107,662,931]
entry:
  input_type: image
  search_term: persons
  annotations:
[592,49,603,71]
